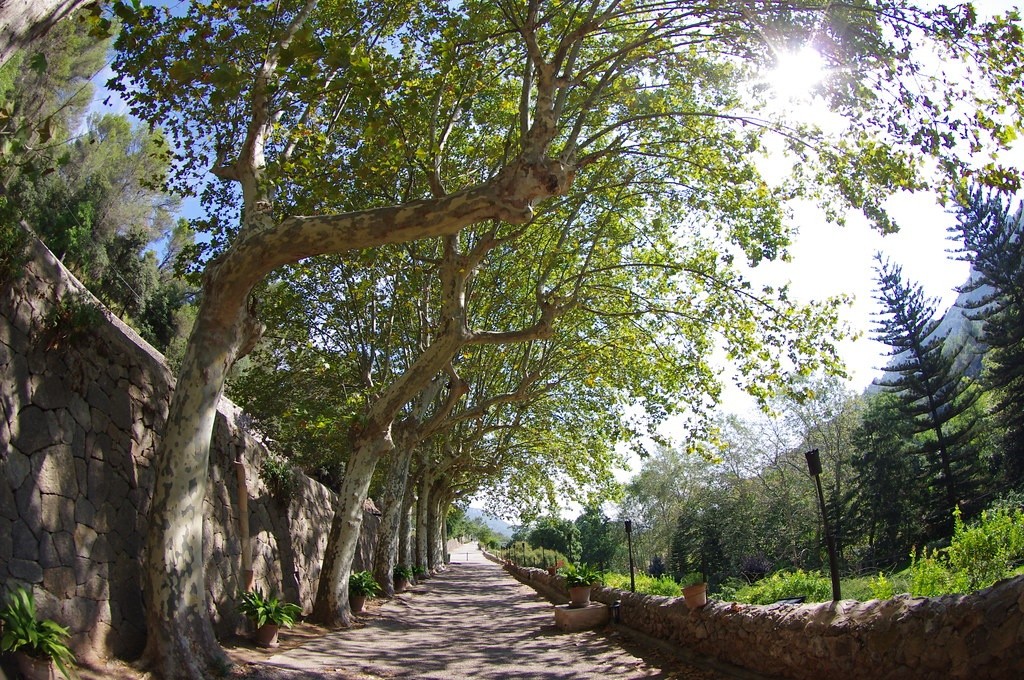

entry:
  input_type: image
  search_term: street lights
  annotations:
[624,520,635,592]
[513,545,516,563]
[804,449,842,601]
[567,533,572,563]
[522,542,525,564]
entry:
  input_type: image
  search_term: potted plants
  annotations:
[563,561,600,607]
[349,569,383,610]
[392,563,412,590]
[231,585,304,648]
[0,583,83,680]
[680,573,707,609]
[412,563,427,581]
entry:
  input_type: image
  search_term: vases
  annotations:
[549,566,557,575]
